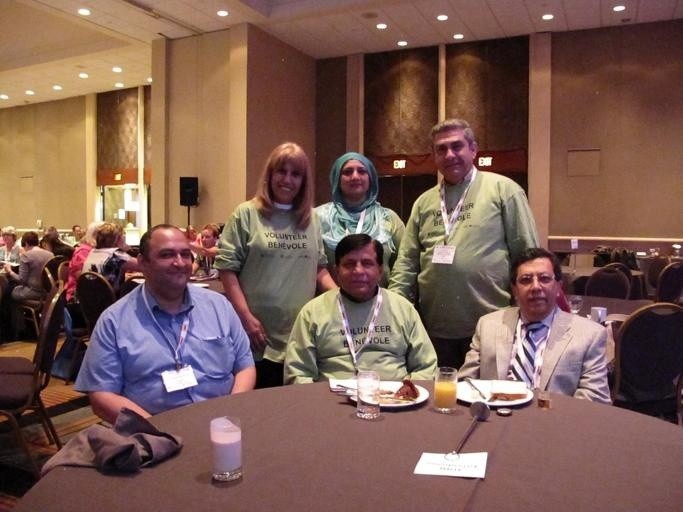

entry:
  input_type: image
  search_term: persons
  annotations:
[387,116,541,382]
[69,224,257,427]
[212,141,340,391]
[282,233,440,386]
[456,247,614,405]
[0,225,141,301]
[185,223,226,276]
[314,151,407,290]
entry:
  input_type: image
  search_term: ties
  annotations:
[512,321,543,384]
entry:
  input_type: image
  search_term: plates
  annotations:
[455,379,533,409]
[348,380,429,409]
[188,283,209,288]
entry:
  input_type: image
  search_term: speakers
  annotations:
[180,177,198,206]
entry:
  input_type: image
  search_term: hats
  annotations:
[1,227,16,235]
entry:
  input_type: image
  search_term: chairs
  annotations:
[610,303,682,426]
[65,272,116,386]
[551,249,683,327]
[57,260,70,286]
[42,255,70,292]
[0,280,68,480]
[44,266,57,290]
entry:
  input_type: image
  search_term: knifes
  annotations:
[463,377,486,399]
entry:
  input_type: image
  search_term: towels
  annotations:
[38,408,183,477]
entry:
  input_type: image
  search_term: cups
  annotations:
[567,295,583,314]
[646,247,659,257]
[208,416,243,484]
[589,306,606,325]
[356,370,382,420]
[36,219,42,229]
[431,366,457,414]
[671,243,680,258]
[537,389,551,409]
